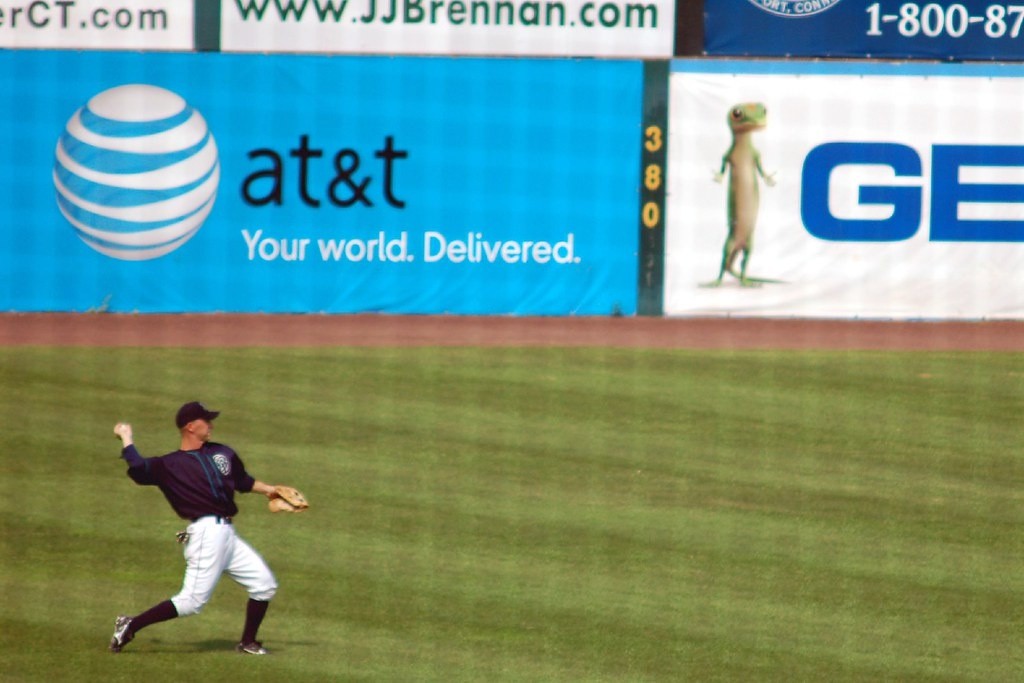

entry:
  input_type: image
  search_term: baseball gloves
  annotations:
[265,486,310,513]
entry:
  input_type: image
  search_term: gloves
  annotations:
[176,530,190,545]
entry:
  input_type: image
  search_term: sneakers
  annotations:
[109,616,134,653]
[238,641,266,655]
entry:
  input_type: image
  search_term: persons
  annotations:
[108,401,310,655]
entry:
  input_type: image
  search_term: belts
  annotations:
[192,515,231,525]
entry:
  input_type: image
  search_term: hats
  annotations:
[175,401,221,428]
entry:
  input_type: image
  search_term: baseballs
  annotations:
[120,424,127,431]
